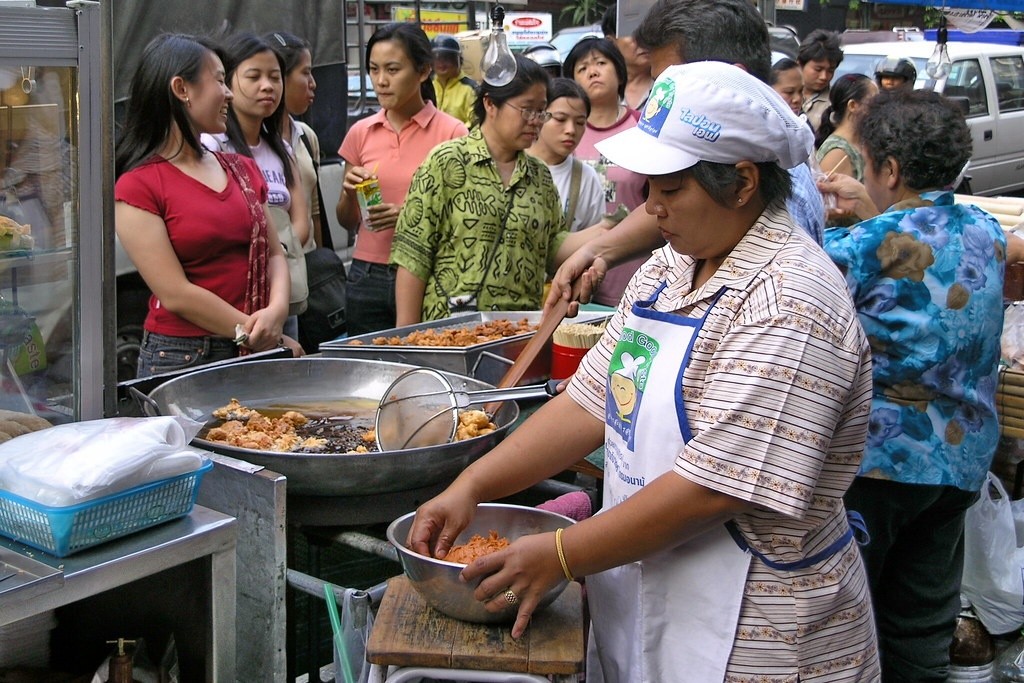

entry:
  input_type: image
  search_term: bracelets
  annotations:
[556,527,585,582]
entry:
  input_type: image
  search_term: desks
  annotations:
[0,503,240,683]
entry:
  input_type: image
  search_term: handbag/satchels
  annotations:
[303,246,350,342]
[962,472,1024,635]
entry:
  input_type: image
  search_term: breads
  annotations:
[1,407,55,445]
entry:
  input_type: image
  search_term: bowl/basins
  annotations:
[386,503,581,622]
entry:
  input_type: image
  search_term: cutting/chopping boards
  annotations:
[364,575,583,672]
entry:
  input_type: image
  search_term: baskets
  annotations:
[1,458,213,556]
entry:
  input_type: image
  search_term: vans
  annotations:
[830,41,1024,200]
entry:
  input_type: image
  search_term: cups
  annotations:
[355,178,383,231]
[551,342,600,379]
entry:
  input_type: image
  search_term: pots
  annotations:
[129,350,538,497]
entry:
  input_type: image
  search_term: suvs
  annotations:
[767,21,801,64]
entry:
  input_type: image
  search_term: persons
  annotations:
[115,0,1024,379]
[814,88,1006,683]
[404,61,882,683]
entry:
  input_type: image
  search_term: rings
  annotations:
[503,590,521,609]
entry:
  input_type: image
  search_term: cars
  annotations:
[346,68,381,127]
[550,21,603,65]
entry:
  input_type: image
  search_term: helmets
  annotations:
[430,34,460,53]
[873,56,916,92]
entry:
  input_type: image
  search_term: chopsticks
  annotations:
[553,316,610,348]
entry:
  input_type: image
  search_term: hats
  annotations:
[523,42,561,67]
[593,60,816,176]
